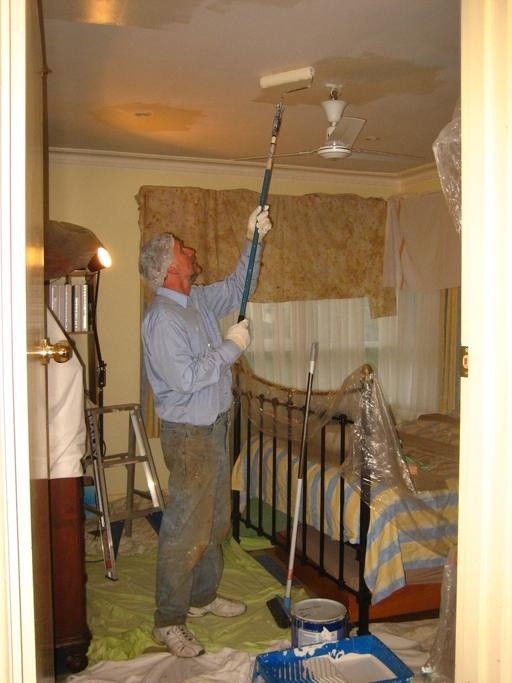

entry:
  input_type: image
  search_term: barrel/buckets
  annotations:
[290,598,347,652]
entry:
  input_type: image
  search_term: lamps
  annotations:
[85,247,113,458]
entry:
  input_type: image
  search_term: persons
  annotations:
[138,205,272,658]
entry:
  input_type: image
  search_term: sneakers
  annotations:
[150,626,205,659]
[186,596,246,619]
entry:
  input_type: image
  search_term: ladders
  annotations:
[86,404,167,581]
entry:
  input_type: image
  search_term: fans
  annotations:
[233,85,424,162]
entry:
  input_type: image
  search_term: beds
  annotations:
[229,352,459,635]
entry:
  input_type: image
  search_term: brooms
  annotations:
[267,342,348,629]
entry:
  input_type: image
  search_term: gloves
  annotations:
[246,205,272,244]
[225,318,252,354]
